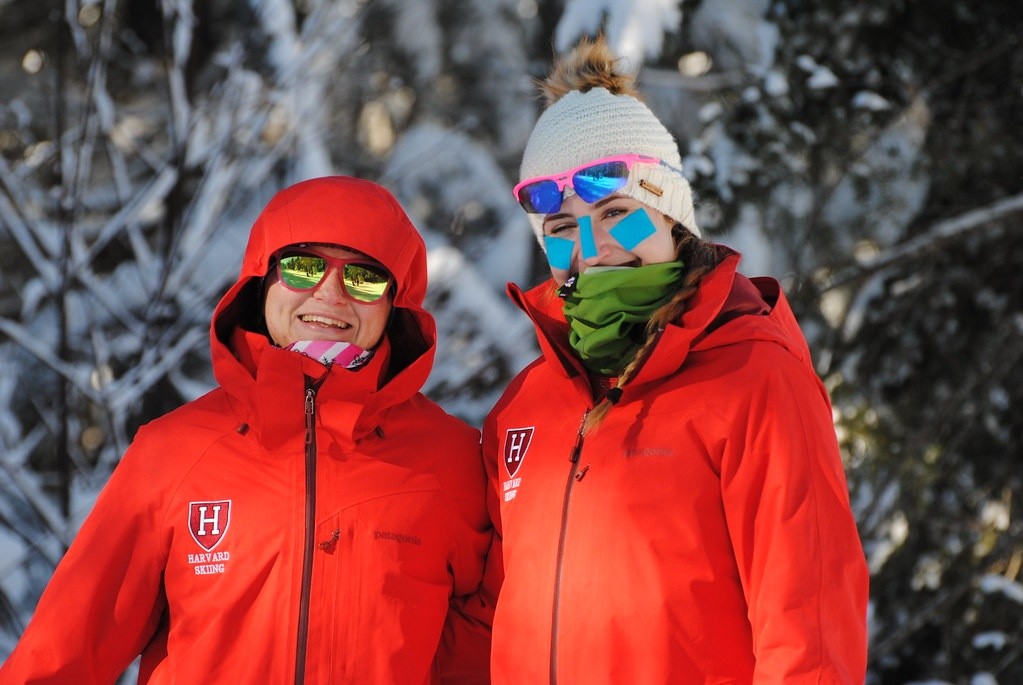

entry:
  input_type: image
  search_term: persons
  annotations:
[0,175,492,685]
[432,37,869,685]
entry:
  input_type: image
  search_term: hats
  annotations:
[516,33,702,255]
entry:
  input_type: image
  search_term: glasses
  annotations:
[260,247,395,305]
[512,154,664,215]
